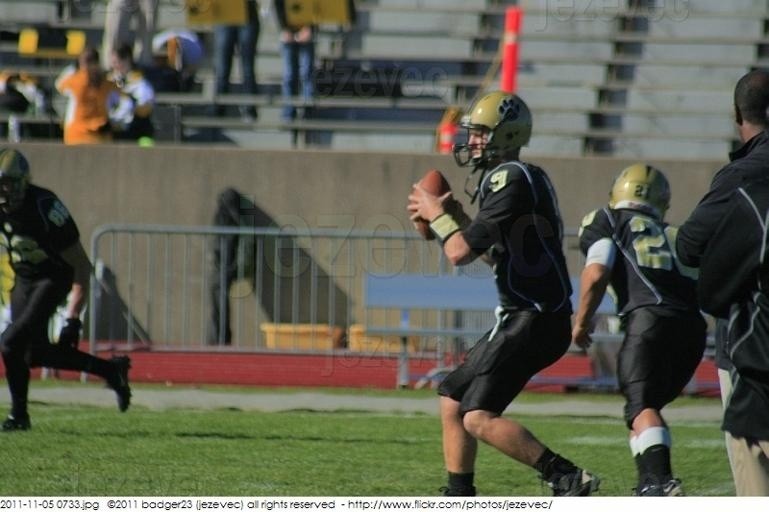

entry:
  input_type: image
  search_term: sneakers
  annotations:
[635,477,685,496]
[0,415,32,432]
[104,355,135,414]
[550,470,602,496]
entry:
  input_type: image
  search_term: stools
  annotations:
[256,321,421,354]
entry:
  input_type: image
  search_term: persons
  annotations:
[54,1,355,149]
[406,92,602,495]
[673,69,768,496]
[570,160,708,496]
[0,147,132,430]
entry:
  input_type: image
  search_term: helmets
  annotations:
[459,91,534,152]
[0,148,30,193]
[607,163,671,221]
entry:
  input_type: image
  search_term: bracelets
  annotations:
[428,212,463,249]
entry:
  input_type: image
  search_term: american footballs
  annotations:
[412,171,452,241]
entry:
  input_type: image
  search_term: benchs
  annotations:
[0,0,769,162]
[363,273,723,397]
